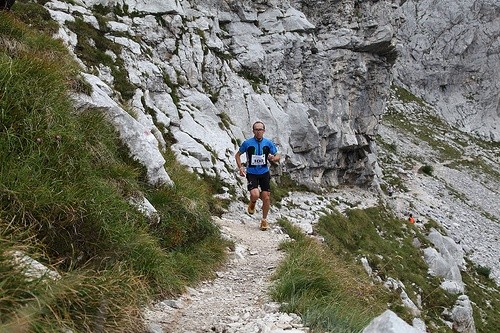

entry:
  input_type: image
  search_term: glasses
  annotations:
[254,129,263,131]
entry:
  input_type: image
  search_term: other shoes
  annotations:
[260,219,268,231]
[248,202,256,214]
